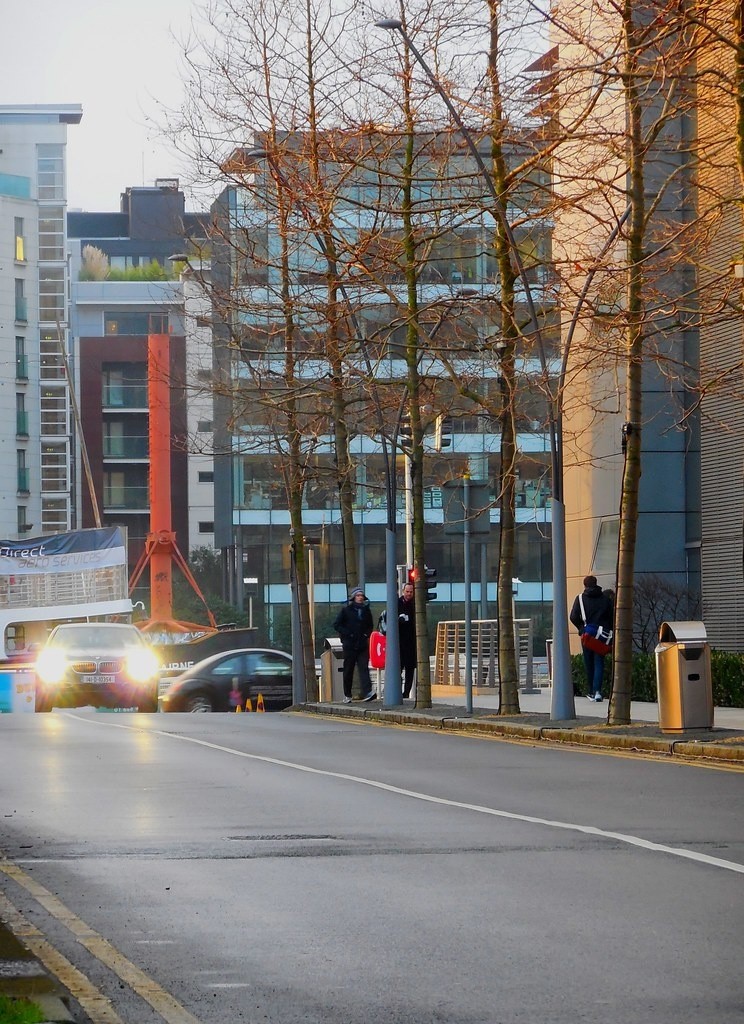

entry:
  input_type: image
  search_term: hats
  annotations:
[341,587,370,606]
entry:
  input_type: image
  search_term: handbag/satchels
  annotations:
[579,624,614,656]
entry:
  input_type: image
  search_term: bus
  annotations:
[0,599,135,714]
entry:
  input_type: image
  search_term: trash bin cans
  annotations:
[320,637,344,703]
[654,620,715,734]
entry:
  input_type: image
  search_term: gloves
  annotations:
[399,614,409,621]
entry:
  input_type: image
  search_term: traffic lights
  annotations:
[423,564,438,605]
[399,411,416,453]
[435,408,454,453]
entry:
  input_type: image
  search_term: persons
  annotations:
[570,576,615,702]
[398,583,418,698]
[333,588,376,704]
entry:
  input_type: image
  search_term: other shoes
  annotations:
[402,694,409,698]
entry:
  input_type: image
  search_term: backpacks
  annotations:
[377,610,387,634]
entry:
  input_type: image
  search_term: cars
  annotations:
[24,622,158,713]
[160,648,319,712]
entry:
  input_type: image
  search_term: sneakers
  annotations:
[365,691,377,702]
[343,696,352,703]
[587,693,595,701]
[595,691,603,702]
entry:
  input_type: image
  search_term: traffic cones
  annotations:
[235,704,243,714]
[245,698,253,712]
[256,691,266,713]
